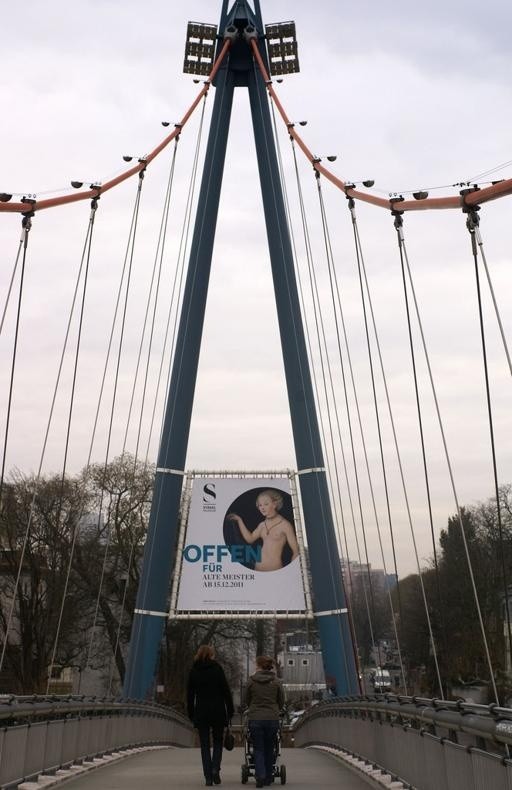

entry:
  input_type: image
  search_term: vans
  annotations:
[367,668,392,693]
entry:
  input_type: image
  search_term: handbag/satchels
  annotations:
[224,726,234,750]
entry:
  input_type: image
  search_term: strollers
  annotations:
[240,713,290,784]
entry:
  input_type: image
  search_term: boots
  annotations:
[203,766,221,786]
[256,776,272,788]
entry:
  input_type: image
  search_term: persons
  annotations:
[239,654,286,788]
[225,489,301,572]
[185,644,235,787]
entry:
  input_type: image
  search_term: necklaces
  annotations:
[264,513,279,520]
[261,516,286,536]
